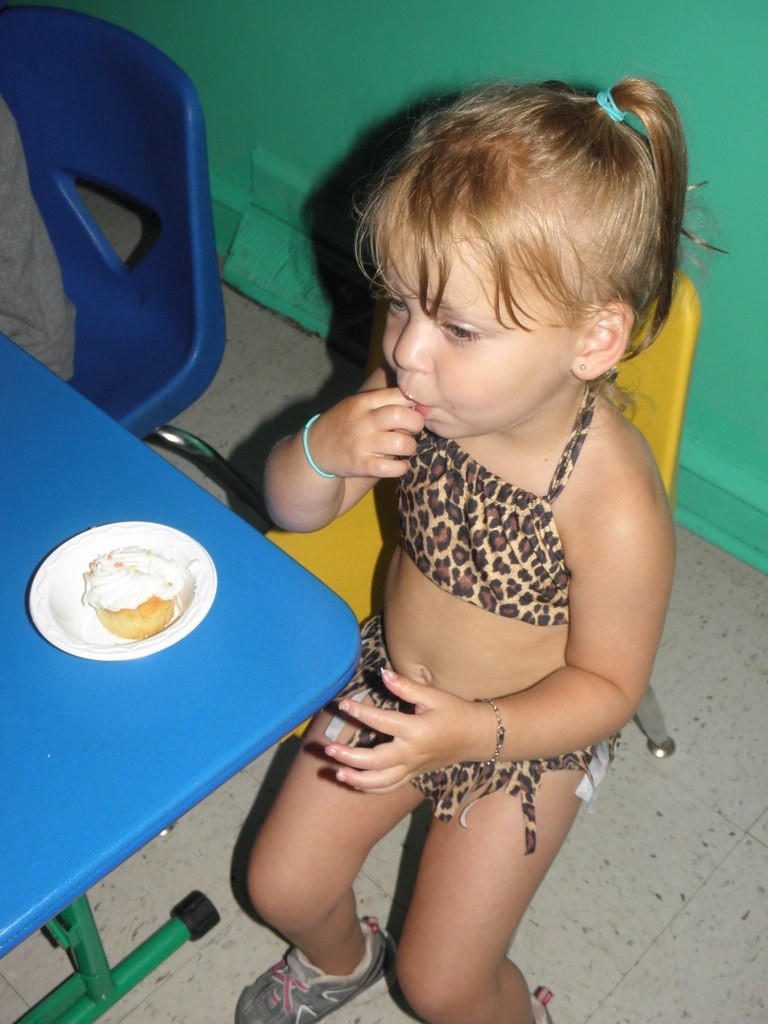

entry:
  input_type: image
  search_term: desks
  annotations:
[0,331,361,1024]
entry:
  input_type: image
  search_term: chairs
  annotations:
[0,6,268,520]
[264,271,701,759]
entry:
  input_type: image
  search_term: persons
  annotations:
[0,95,77,383]
[234,78,731,1023]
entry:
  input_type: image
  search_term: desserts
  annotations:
[82,547,196,638]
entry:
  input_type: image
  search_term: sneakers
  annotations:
[236,916,397,1024]
[529,985,554,1024]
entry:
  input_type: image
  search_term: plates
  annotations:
[27,520,218,663]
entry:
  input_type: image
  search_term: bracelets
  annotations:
[303,413,335,478]
[473,698,506,766]
